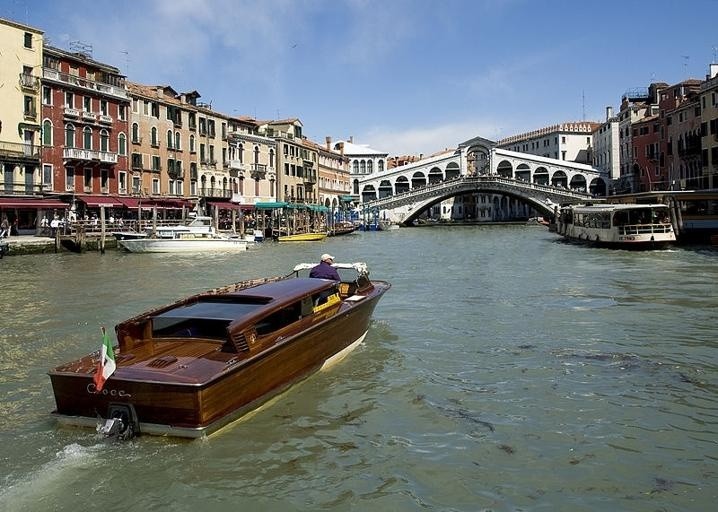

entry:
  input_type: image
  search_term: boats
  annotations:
[119,232,248,252]
[278,233,327,241]
[603,188,718,244]
[330,228,355,235]
[527,217,543,225]
[556,201,676,249]
[144,216,241,239]
[413,217,448,224]
[379,220,399,231]
[46,262,393,439]
[111,231,256,246]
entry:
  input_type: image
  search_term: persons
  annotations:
[1,203,377,238]
[309,253,341,298]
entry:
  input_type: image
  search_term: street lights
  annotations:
[136,178,144,231]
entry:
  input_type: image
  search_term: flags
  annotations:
[92,330,117,392]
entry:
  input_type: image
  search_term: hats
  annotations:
[320,253,336,262]
